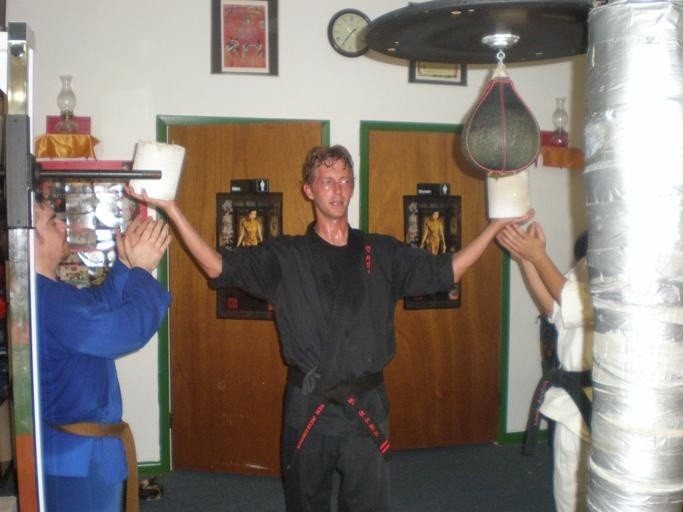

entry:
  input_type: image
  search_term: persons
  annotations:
[496,220,595,511]
[421,209,448,256]
[123,144,535,511]
[32,191,173,511]
[235,208,265,248]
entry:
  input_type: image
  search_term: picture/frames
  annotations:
[211,0,279,75]
[408,58,468,86]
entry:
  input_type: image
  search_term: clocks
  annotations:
[327,8,372,57]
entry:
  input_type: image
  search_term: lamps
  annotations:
[54,76,79,133]
[550,97,570,147]
